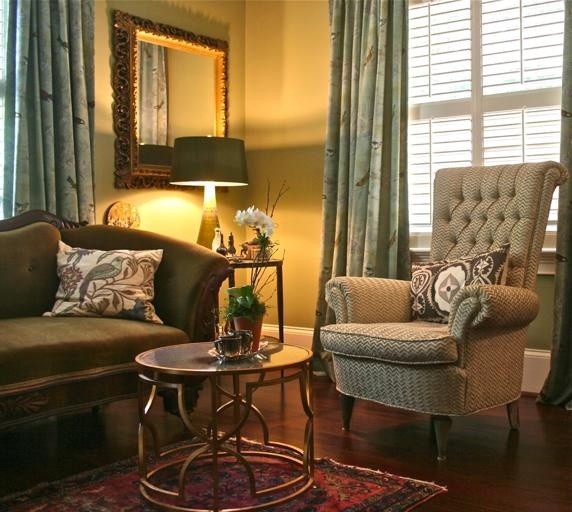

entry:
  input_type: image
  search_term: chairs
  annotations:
[317,160,568,463]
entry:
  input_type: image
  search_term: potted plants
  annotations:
[203,244,289,353]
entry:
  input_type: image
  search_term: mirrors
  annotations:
[108,7,231,195]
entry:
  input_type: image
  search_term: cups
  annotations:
[214,336,242,361]
[230,330,253,355]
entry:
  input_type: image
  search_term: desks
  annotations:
[227,259,287,378]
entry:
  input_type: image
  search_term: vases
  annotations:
[243,243,278,261]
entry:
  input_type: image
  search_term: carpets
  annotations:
[0,430,450,512]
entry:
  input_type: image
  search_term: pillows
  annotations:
[408,240,513,325]
[0,219,63,320]
[40,239,166,326]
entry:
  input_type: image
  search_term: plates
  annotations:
[107,201,141,228]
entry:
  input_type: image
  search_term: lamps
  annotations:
[139,143,174,166]
[168,136,250,249]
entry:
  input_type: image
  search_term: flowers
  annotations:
[233,173,293,246]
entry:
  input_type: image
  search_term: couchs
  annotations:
[0,208,237,429]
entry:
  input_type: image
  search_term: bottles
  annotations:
[211,226,236,258]
